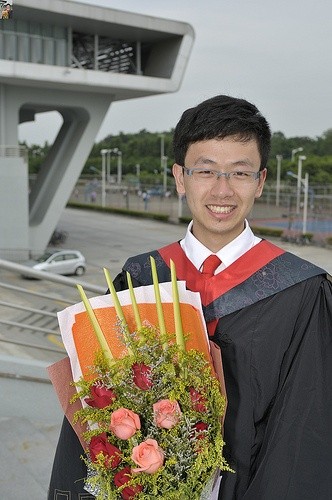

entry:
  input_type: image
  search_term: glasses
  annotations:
[182,166,261,185]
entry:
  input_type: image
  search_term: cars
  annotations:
[22,249,86,280]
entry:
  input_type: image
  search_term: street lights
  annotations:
[276,147,311,235]
[100,148,125,209]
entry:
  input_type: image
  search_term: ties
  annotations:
[201,254,223,280]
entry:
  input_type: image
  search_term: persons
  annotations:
[46,95,332,500]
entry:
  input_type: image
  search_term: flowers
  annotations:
[45,256,236,500]
